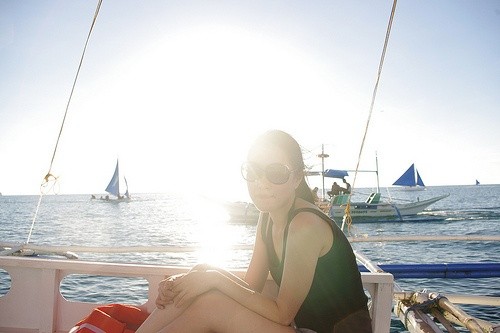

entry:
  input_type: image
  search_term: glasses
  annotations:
[240,160,300,185]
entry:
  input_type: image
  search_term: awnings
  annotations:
[323,169,349,178]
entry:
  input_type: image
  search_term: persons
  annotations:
[134,130,373,333]
[90,194,125,202]
[311,178,352,200]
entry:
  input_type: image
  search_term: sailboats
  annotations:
[226,142,451,223]
[91,158,132,203]
[392,163,427,191]
